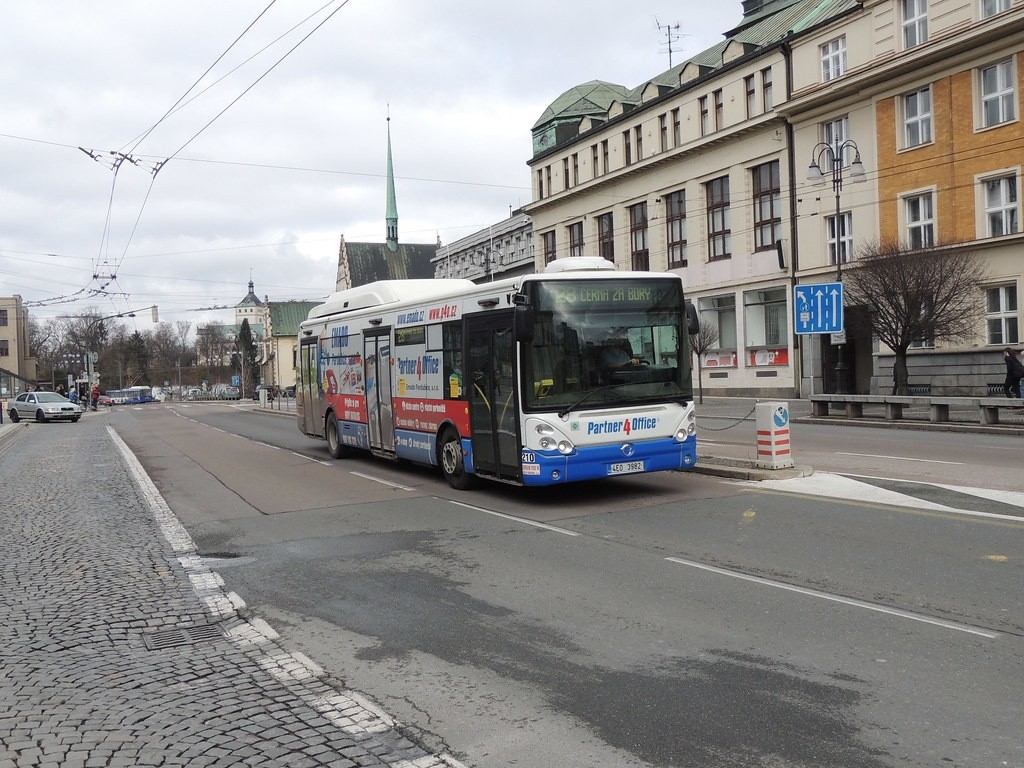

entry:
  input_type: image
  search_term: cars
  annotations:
[6,391,83,423]
[97,395,114,406]
[187,383,239,398]
[252,385,275,401]
[283,386,295,396]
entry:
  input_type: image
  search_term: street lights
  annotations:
[805,133,866,410]
[85,305,159,411]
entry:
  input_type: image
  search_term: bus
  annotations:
[296,255,702,490]
[106,386,152,404]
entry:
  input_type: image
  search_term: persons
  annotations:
[25,380,90,410]
[1003,347,1022,409]
[91,383,99,411]
[473,367,486,397]
[1020,351,1024,397]
[494,369,503,396]
[450,352,465,397]
[601,334,640,369]
